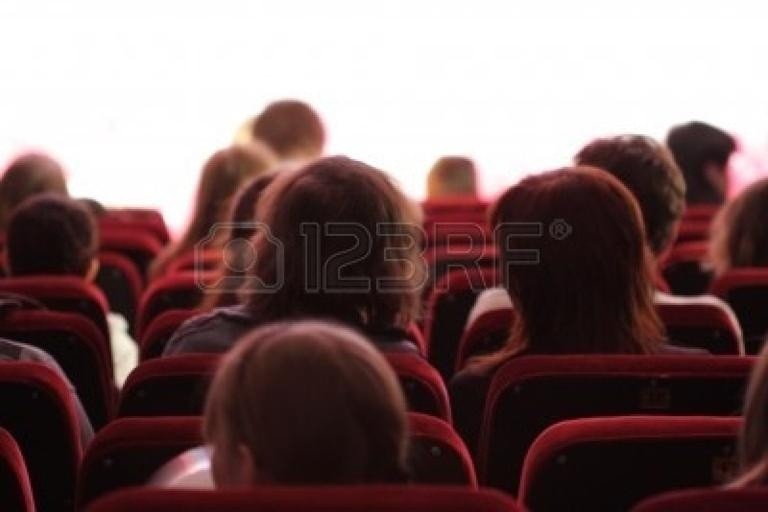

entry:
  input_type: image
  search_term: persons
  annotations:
[0,150,73,224]
[200,316,408,491]
[201,176,275,308]
[2,193,101,284]
[666,120,742,204]
[426,155,477,201]
[251,100,325,165]
[156,146,269,263]
[158,156,423,357]
[712,340,767,489]
[571,133,687,270]
[722,174,768,268]
[446,163,713,422]
